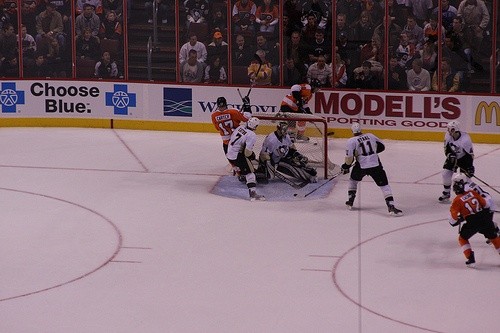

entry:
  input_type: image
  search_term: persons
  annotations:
[339,122,405,213]
[448,171,500,265]
[210,96,250,176]
[0,0,171,79]
[177,0,500,93]
[255,121,318,183]
[279,77,324,144]
[226,116,267,202]
[451,167,500,246]
[438,119,476,203]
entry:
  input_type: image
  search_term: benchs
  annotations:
[125,0,230,84]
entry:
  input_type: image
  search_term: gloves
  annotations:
[341,162,351,174]
[305,107,313,114]
[242,96,251,105]
[443,158,454,171]
[448,153,458,168]
[247,151,256,160]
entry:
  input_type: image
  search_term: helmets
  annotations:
[291,84,301,91]
[447,120,461,131]
[453,181,465,190]
[217,97,227,107]
[277,121,288,132]
[310,78,324,89]
[453,172,468,182]
[248,117,260,129]
[352,122,361,133]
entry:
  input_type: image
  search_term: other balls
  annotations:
[314,143,317,145]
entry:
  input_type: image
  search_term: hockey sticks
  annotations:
[301,108,335,136]
[254,159,310,189]
[492,210,500,214]
[448,157,500,195]
[239,53,263,113]
[293,163,356,198]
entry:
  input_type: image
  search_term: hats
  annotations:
[213,32,222,37]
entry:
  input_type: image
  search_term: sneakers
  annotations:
[346,198,354,209]
[233,169,247,184]
[248,189,265,201]
[289,132,296,143]
[486,239,493,245]
[297,135,310,143]
[388,206,403,217]
[465,251,476,267]
[438,191,451,204]
